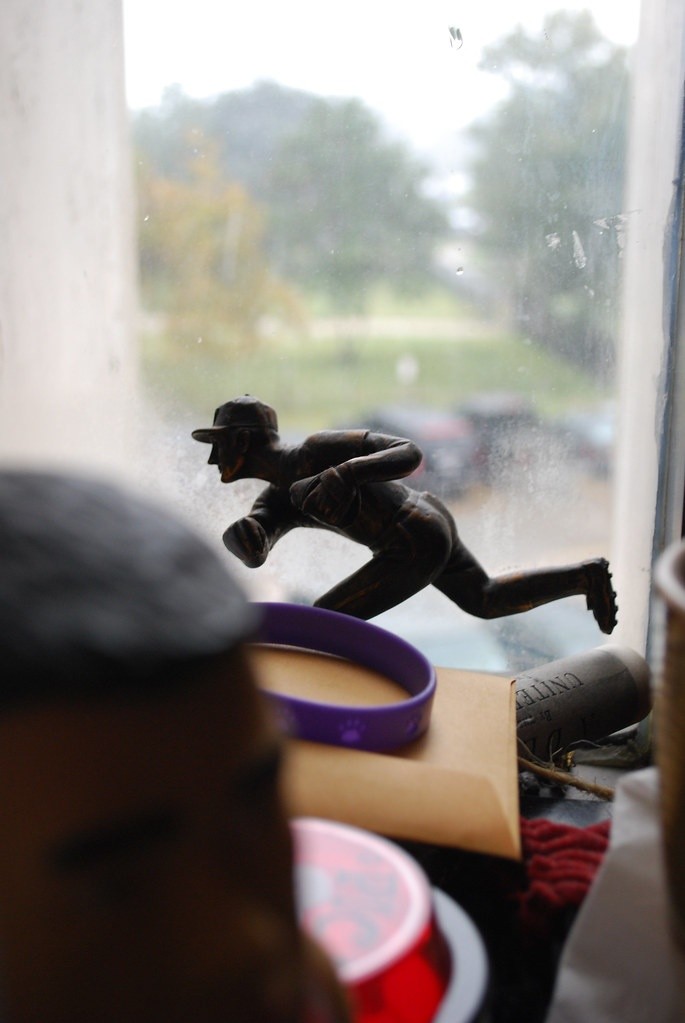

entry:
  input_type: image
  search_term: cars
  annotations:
[359,382,618,494]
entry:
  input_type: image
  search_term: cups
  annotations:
[653,539,685,900]
[293,819,451,1023]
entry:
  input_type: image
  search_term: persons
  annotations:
[0,464,344,1023]
[191,393,618,634]
